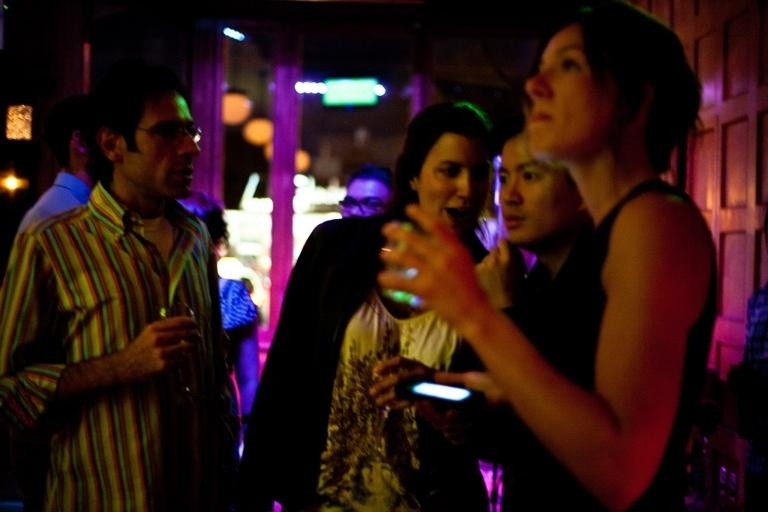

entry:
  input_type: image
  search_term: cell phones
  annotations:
[395,373,486,415]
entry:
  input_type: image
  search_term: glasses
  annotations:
[137,125,202,143]
[340,200,386,214]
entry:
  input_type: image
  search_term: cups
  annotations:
[162,305,198,400]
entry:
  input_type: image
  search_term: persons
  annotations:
[381,2,717,510]
[241,104,591,511]
[1,53,241,511]
